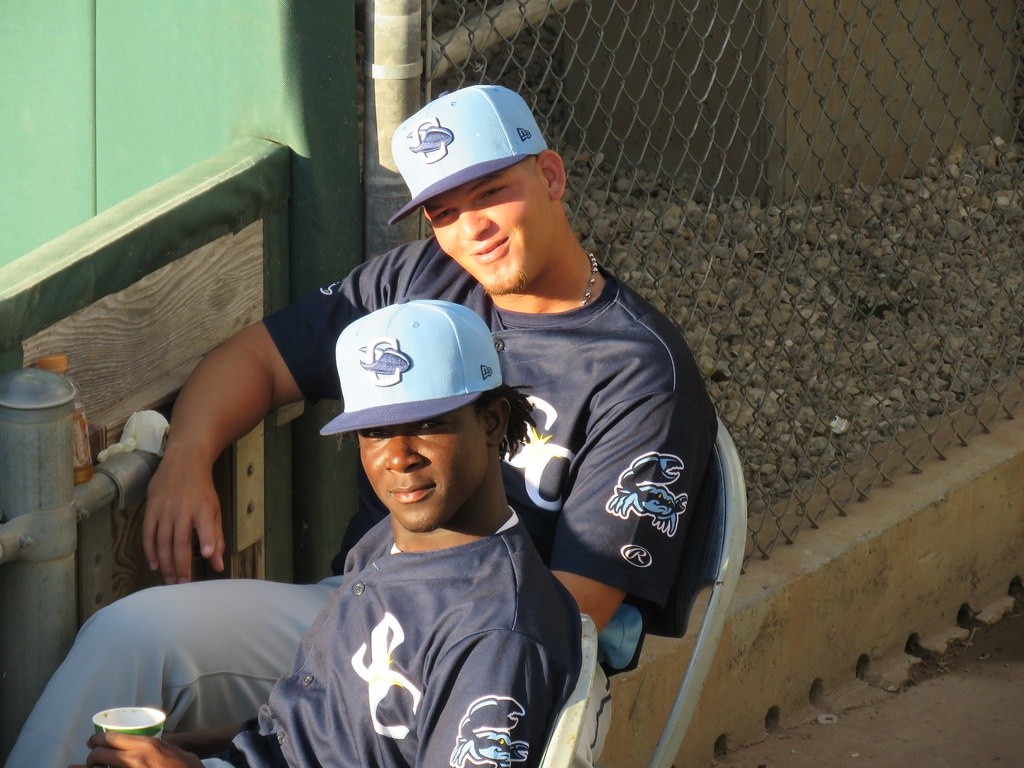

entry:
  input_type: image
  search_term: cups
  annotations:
[92,708,166,768]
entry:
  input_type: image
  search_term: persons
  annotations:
[0,85,718,768]
[85,300,583,768]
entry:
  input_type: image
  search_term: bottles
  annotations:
[38,357,93,483]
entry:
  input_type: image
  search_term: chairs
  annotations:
[647,415,747,768]
[538,613,598,768]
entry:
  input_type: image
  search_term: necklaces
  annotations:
[582,249,597,305]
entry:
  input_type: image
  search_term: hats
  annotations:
[385,84,550,227]
[319,297,503,437]
